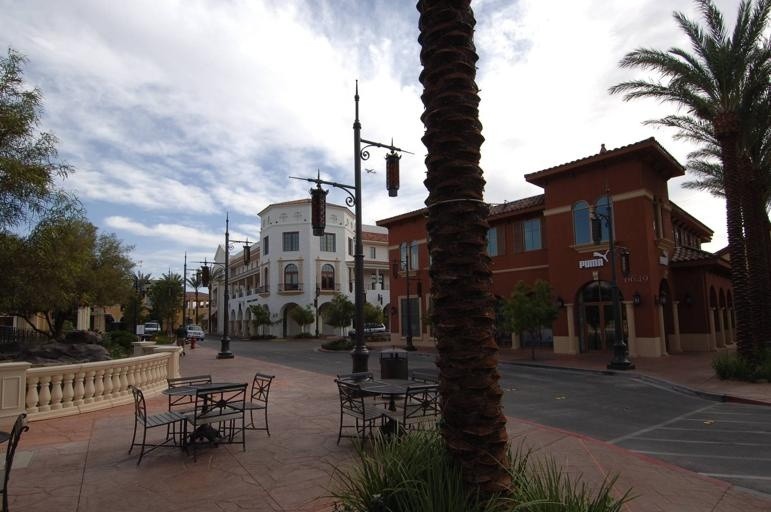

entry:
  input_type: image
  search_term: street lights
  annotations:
[591,186,636,369]
[286,78,418,394]
[389,255,422,352]
[181,250,204,346]
[132,276,151,336]
[199,209,250,359]
[313,275,321,338]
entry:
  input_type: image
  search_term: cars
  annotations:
[143,322,160,334]
[186,325,204,342]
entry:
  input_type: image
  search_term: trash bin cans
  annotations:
[379,346,408,379]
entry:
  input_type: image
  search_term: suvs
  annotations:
[348,319,385,334]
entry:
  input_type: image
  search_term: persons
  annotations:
[176,324,187,356]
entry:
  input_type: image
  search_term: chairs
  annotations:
[333,371,444,452]
[0,413,29,512]
[127,371,276,465]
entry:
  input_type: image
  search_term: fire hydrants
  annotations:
[189,335,197,350]
